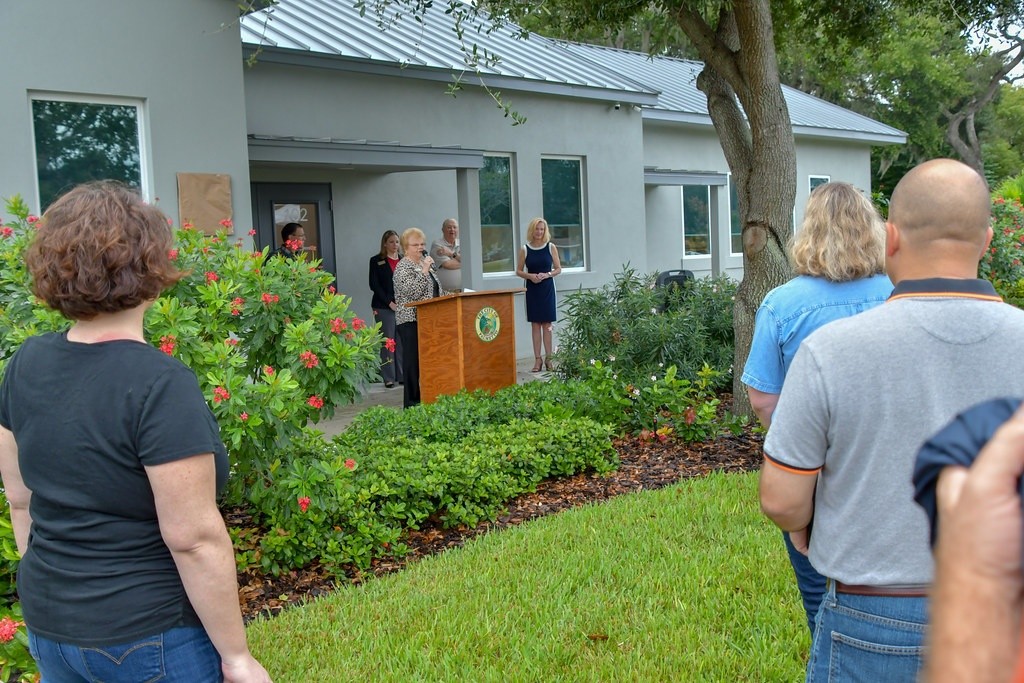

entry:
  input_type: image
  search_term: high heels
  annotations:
[532,356,543,372]
[545,357,552,371]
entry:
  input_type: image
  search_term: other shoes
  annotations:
[385,381,394,388]
[399,381,404,385]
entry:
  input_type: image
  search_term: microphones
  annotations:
[422,250,438,272]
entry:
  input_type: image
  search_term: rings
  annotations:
[429,260,430,261]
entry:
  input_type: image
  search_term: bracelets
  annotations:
[548,272,551,276]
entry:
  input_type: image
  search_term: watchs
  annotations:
[453,252,457,258]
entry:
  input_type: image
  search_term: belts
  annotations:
[826,577,931,597]
[447,289,461,293]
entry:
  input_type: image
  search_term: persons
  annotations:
[742,181,895,633]
[266,223,306,263]
[0,181,273,683]
[918,406,1024,683]
[430,218,461,294]
[369,231,405,388]
[392,228,453,409]
[516,217,561,372]
[760,157,1024,683]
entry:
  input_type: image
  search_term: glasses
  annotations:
[291,234,307,239]
[407,242,426,248]
